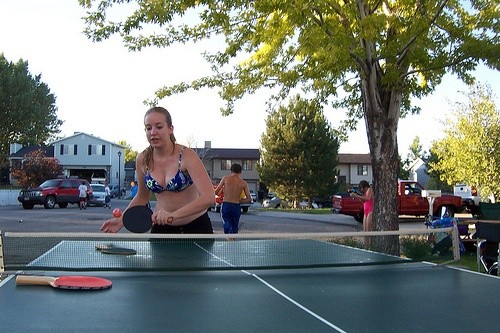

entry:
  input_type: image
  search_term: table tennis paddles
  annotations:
[14,274,113,292]
[95,241,138,257]
[122,203,175,234]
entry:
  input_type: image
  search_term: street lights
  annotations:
[117,151,122,199]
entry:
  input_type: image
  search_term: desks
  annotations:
[0,240,500,333]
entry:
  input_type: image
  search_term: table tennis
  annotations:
[112,207,124,219]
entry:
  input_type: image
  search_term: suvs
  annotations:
[17,174,94,209]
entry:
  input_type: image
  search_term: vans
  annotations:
[452,180,497,213]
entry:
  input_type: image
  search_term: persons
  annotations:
[426,211,464,257]
[103,184,112,209]
[100,107,216,241]
[78,181,87,210]
[349,180,374,246]
[215,164,251,234]
[131,181,138,199]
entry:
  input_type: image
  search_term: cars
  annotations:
[207,185,252,214]
[250,192,256,202]
[88,183,111,207]
[108,184,126,198]
[263,190,364,210]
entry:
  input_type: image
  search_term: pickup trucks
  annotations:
[329,178,465,224]
